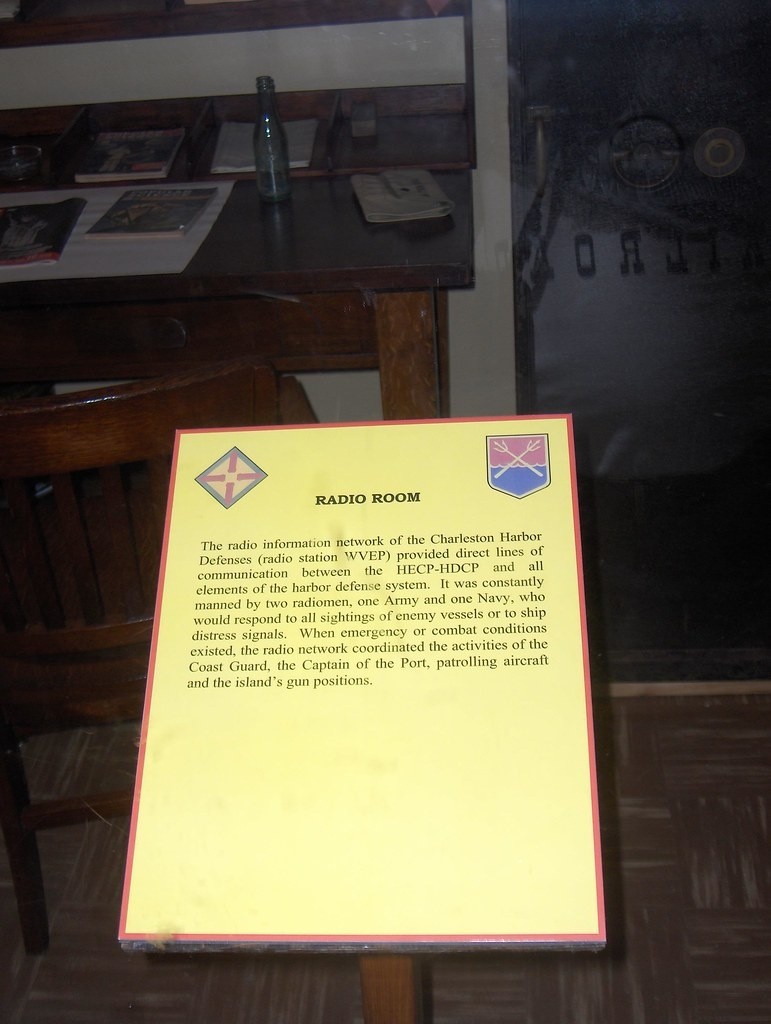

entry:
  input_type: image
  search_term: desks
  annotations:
[1,167,474,417]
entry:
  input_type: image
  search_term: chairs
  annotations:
[1,357,326,958]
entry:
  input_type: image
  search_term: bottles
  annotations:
[251,74,294,203]
